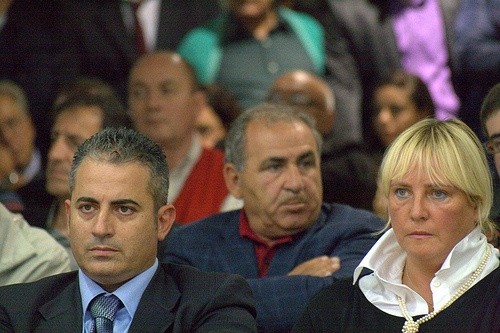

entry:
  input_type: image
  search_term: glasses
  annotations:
[482,136,500,155]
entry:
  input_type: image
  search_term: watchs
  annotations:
[0,167,20,193]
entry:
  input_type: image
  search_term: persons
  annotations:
[0,1,500,136]
[370,69,435,151]
[158,100,392,333]
[0,126,259,333]
[44,82,129,272]
[479,77,500,206]
[0,197,73,287]
[120,50,246,233]
[0,83,55,233]
[255,70,379,211]
[292,116,500,333]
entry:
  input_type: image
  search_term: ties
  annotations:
[88,292,126,333]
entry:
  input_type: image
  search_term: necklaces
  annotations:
[395,239,492,332]
